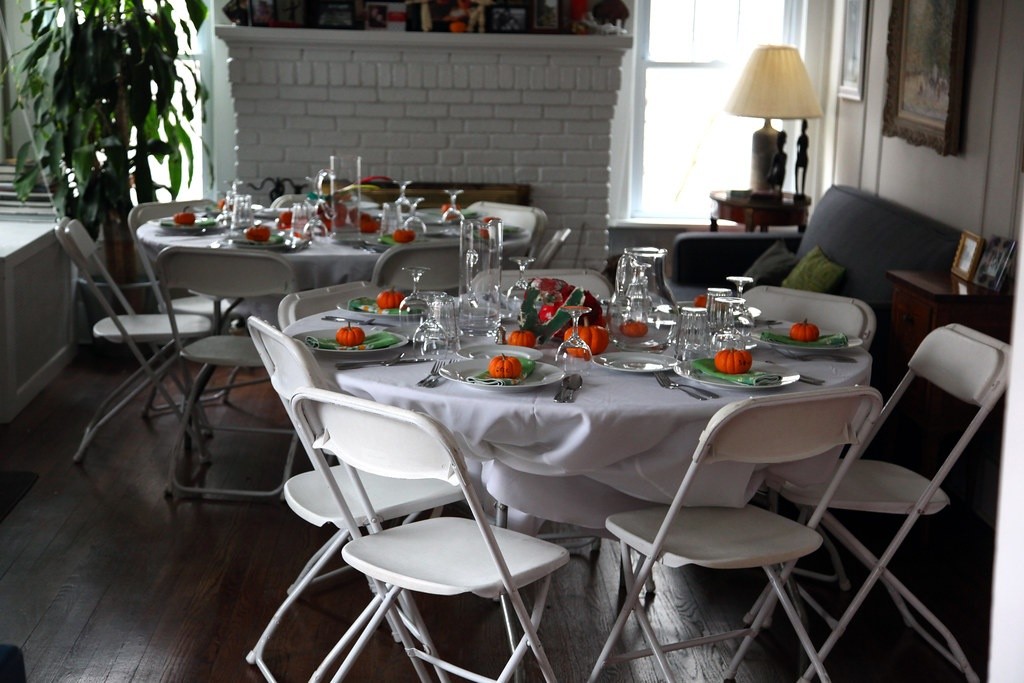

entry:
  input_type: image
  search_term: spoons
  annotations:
[322,317,374,324]
[563,373,583,404]
[393,180,465,236]
[339,352,405,370]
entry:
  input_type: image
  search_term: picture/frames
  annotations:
[836,0,867,101]
[882,1,972,155]
[530,0,569,34]
[484,5,528,34]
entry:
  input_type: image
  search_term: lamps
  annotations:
[708,42,822,231]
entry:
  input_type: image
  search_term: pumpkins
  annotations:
[487,352,523,379]
[334,320,366,348]
[694,294,709,307]
[507,323,535,348]
[788,318,820,341]
[620,318,649,339]
[712,344,753,374]
[376,286,404,310]
[174,185,507,249]
[564,316,611,357]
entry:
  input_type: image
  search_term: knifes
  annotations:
[553,376,571,402]
[320,314,395,329]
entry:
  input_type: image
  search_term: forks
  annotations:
[417,356,457,388]
[653,369,719,401]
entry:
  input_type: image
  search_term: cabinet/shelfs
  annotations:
[0,218,73,428]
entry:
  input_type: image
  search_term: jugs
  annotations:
[605,247,680,351]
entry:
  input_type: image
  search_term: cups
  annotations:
[673,288,751,364]
[290,202,313,238]
[456,218,504,336]
[332,154,363,243]
[229,194,253,234]
[381,202,402,237]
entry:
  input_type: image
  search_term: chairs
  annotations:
[48,175,1012,683]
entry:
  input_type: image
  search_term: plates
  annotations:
[593,352,680,373]
[156,206,293,249]
[438,344,565,393]
[673,358,800,392]
[335,302,426,318]
[750,328,864,355]
[292,329,408,354]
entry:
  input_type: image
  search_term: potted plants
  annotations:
[12,1,209,370]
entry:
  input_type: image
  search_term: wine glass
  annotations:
[555,306,594,376]
[413,289,448,360]
[397,265,431,331]
[506,256,535,316]
